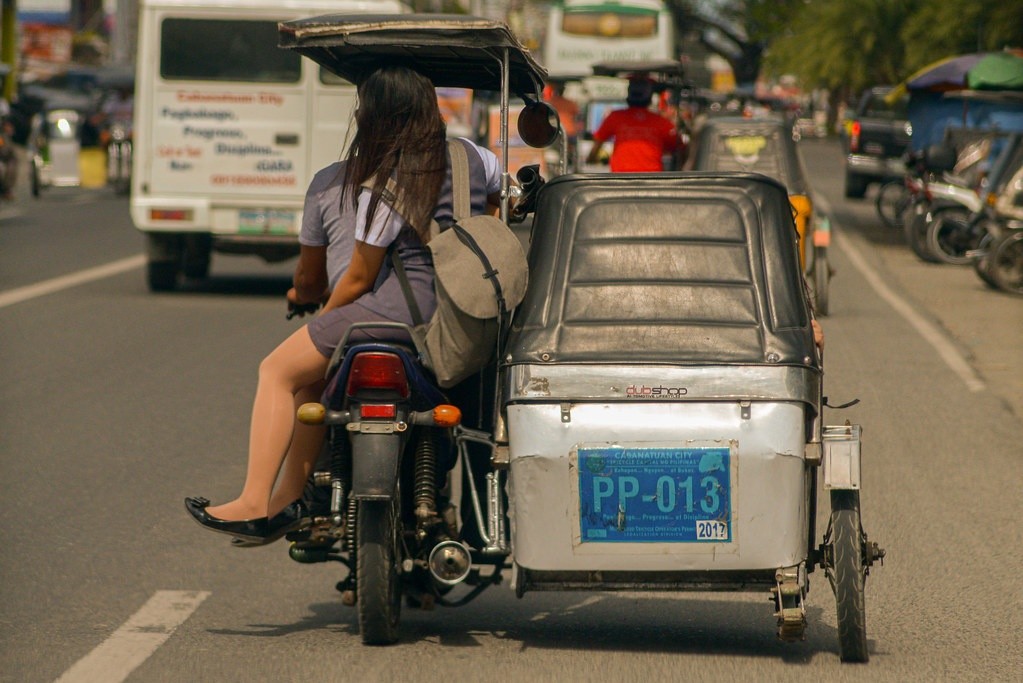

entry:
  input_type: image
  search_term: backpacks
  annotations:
[362,134,529,389]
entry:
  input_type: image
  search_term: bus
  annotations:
[542,0,675,78]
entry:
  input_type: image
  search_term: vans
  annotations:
[127,0,419,292]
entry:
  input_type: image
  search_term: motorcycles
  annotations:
[902,119,1023,296]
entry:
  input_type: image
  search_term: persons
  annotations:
[287,70,528,318]
[586,74,687,173]
[186,65,486,548]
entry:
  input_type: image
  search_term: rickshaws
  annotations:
[284,11,888,663]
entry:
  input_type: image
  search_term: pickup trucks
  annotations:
[842,86,913,198]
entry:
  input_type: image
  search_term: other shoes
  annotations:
[231,498,313,547]
[185,496,268,545]
